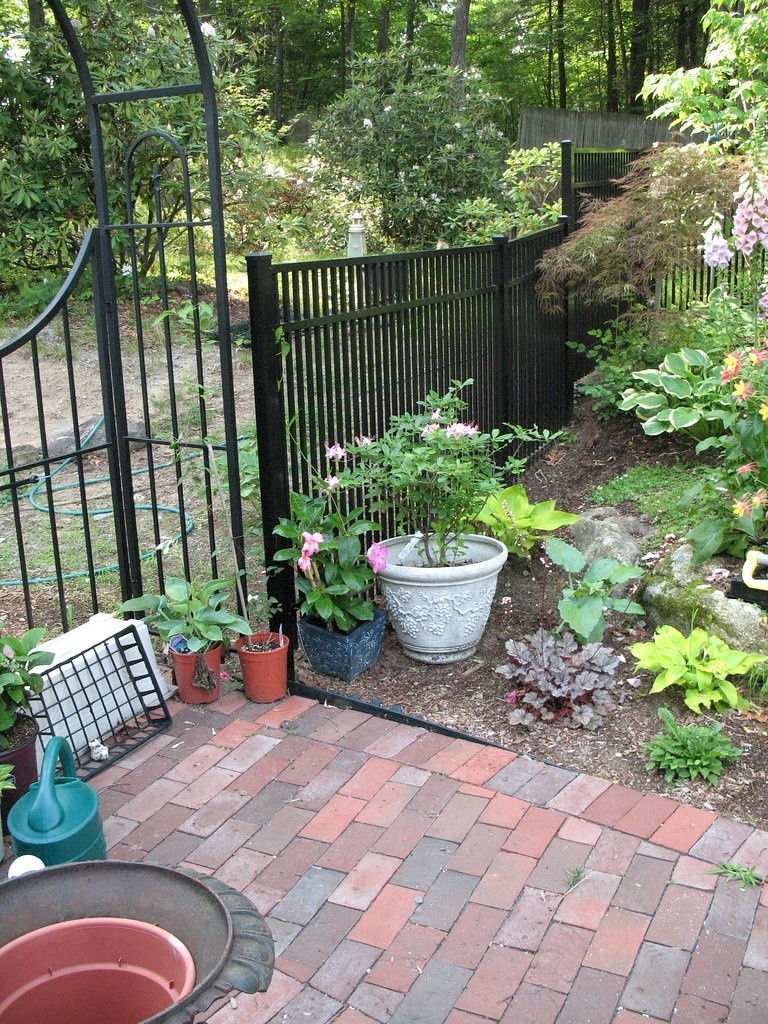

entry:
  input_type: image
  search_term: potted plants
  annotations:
[364,248,405,294]
[0,621,56,835]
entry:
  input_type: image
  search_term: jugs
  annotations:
[6,736,108,879]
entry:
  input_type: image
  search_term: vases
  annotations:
[368,532,509,665]
[169,643,223,702]
[234,631,290,703]
[294,609,387,681]
[0,916,195,1024]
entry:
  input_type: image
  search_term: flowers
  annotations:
[271,410,391,632]
[236,521,285,652]
[116,534,254,655]
[316,376,578,567]
